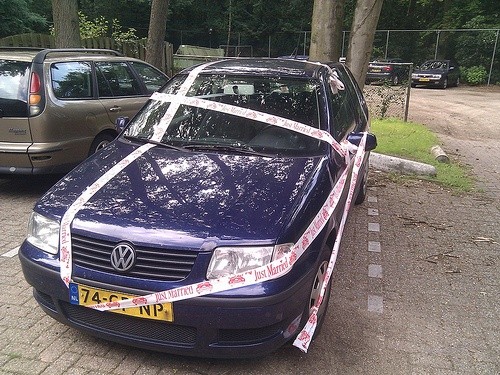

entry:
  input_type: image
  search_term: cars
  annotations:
[364,57,414,86]
[409,59,462,91]
[17,57,378,361]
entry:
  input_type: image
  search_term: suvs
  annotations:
[0,45,170,178]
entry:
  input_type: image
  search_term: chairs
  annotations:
[199,94,255,144]
[99,71,128,96]
[275,91,317,132]
